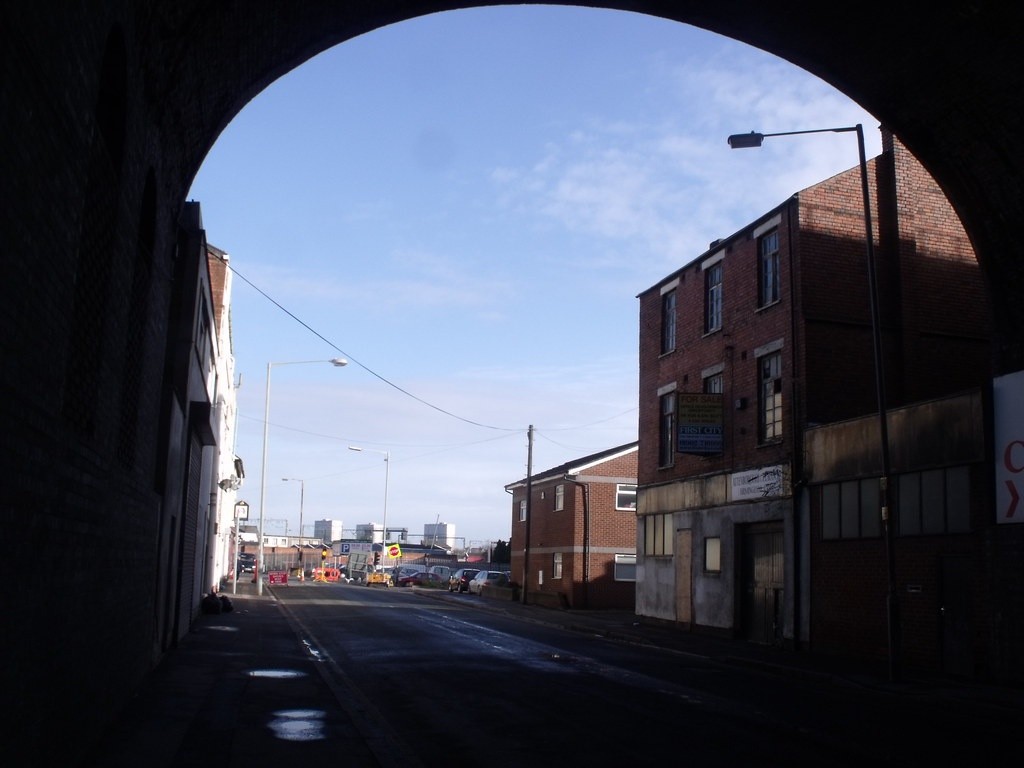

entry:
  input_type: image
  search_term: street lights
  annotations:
[281,476,305,572]
[348,447,391,580]
[256,358,349,594]
[727,125,911,723]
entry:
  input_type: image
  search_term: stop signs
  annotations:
[387,544,402,559]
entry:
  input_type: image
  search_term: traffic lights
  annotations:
[373,552,380,565]
[322,547,327,560]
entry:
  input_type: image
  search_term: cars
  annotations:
[325,563,345,576]
[398,572,444,588]
[393,568,417,584]
[468,571,509,597]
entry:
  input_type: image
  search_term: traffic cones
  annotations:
[298,568,305,581]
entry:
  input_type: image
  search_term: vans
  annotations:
[429,566,449,581]
[239,553,257,573]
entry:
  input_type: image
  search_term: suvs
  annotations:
[448,568,481,593]
